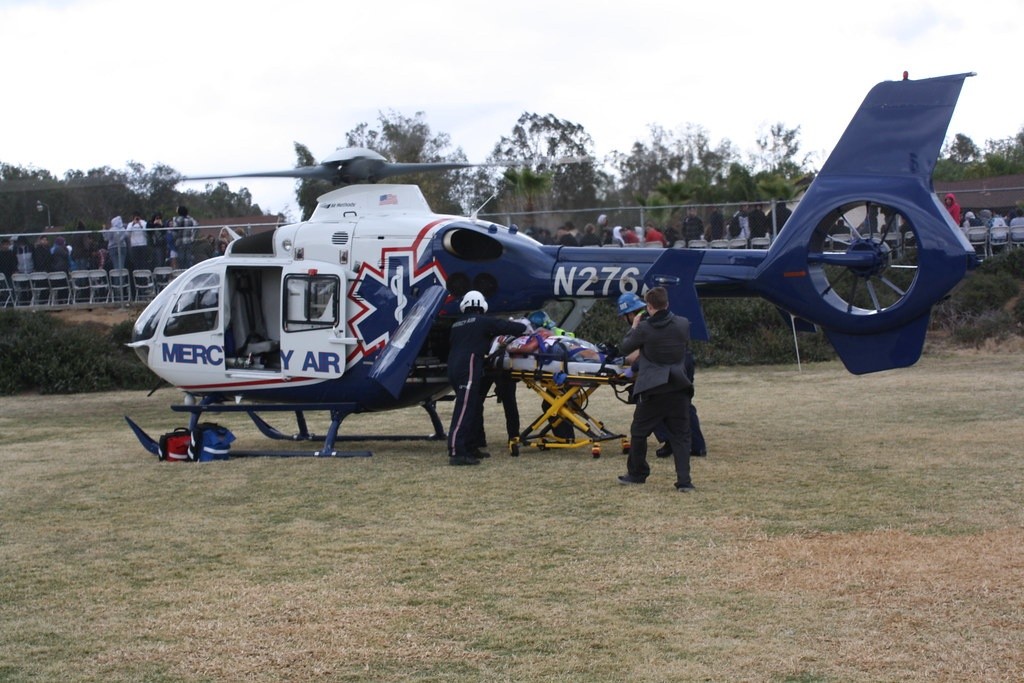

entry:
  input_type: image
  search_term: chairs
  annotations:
[584,222,1024,263]
[173,268,189,278]
[154,266,174,297]
[233,282,280,360]
[132,269,155,301]
[107,268,132,303]
[30,271,51,305]
[70,270,93,304]
[0,272,13,308]
[10,274,32,305]
[48,272,71,305]
[88,269,112,303]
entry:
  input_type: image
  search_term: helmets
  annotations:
[616,293,647,317]
[460,290,488,314]
[528,311,550,324]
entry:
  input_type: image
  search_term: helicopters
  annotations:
[0,70,991,460]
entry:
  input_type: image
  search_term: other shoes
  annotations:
[690,448,707,456]
[656,441,673,457]
[617,475,645,486]
[677,484,695,492]
[450,448,490,466]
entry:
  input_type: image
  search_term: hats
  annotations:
[597,214,608,227]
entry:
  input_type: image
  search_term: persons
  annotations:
[524,191,1024,249]
[617,292,707,456]
[529,311,574,441]
[0,206,247,304]
[472,375,519,445]
[616,287,695,489]
[499,326,639,364]
[448,290,526,464]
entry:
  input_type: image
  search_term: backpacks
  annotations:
[729,213,744,237]
